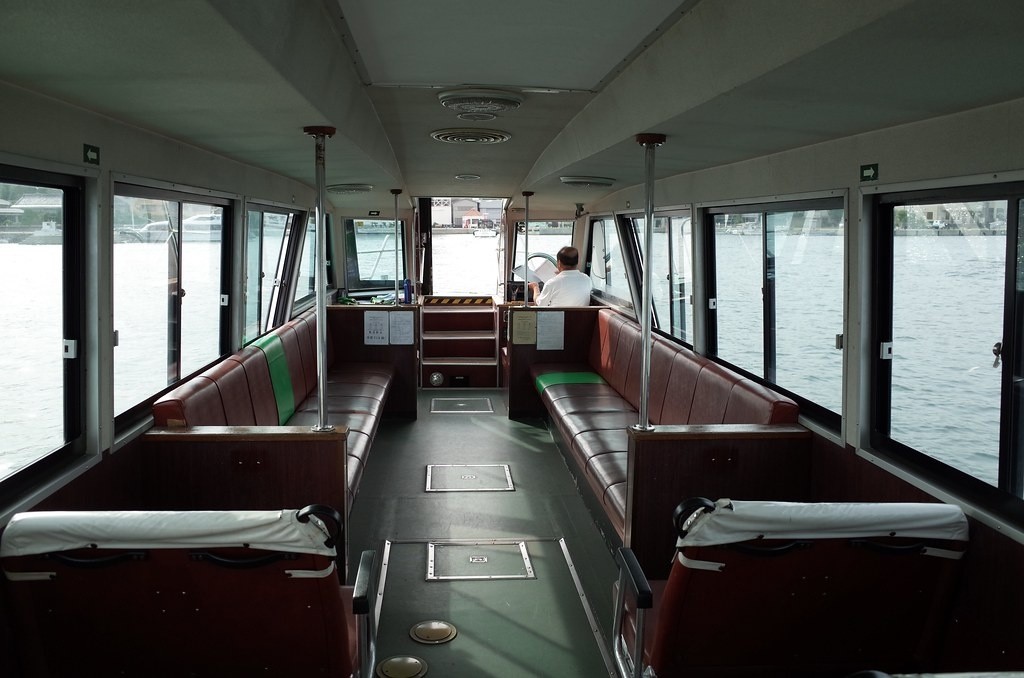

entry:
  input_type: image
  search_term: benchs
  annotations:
[0,504,377,678]
[507,305,811,580]
[144,303,420,585]
[611,498,970,678]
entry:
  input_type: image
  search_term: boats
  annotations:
[473,227,496,238]
[135,208,257,243]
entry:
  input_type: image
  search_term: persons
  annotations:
[528,247,593,306]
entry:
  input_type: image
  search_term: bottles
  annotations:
[404,279,412,304]
